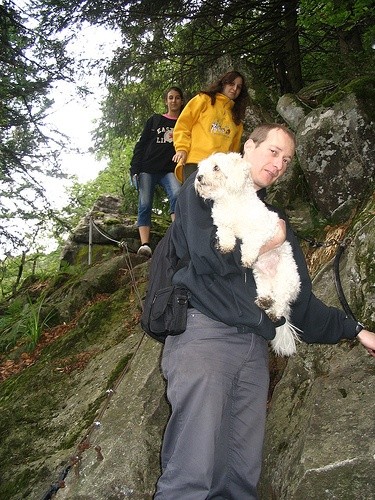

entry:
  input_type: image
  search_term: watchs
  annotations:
[354,321,365,335]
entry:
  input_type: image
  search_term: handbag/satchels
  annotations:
[148,283,189,336]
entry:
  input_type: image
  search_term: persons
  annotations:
[173,69,250,188]
[129,86,182,257]
[154,122,375,500]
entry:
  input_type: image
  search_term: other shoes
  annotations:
[137,243,152,259]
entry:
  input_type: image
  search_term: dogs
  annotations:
[194,151,302,356]
